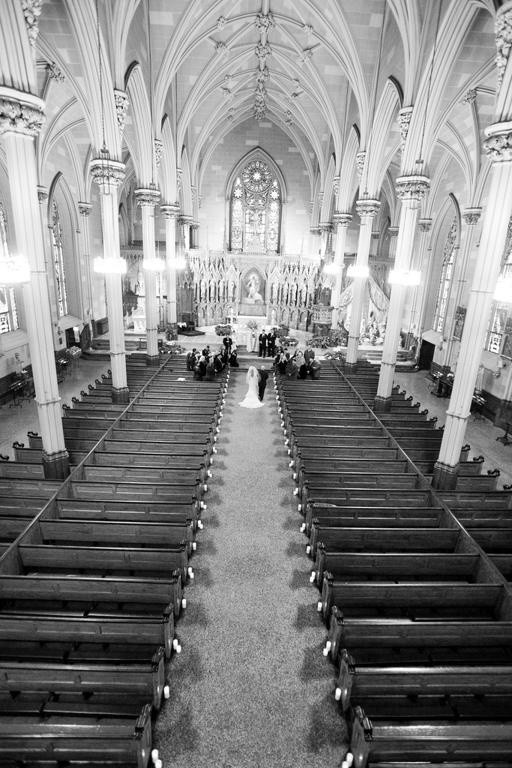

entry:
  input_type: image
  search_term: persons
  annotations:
[246,276,260,298]
[186,326,322,409]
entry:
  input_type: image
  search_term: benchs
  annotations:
[295,446,510,767]
[61,352,232,446]
[276,359,455,488]
[1,358,64,409]
[2,429,217,768]
[472,386,512,447]
